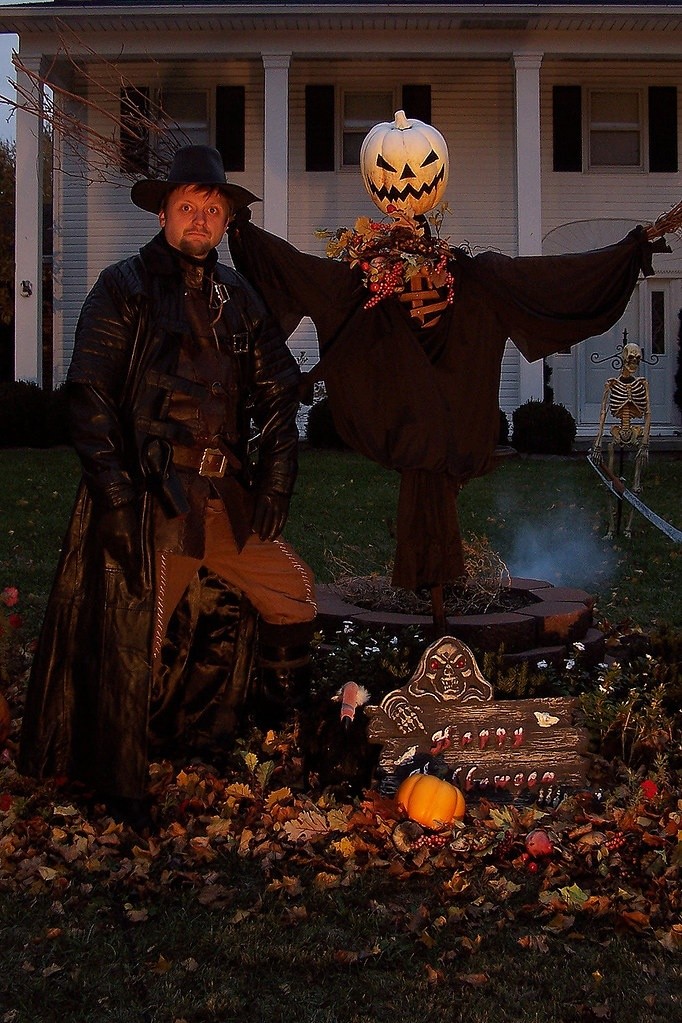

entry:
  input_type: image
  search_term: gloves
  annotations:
[251,487,292,542]
[93,504,139,582]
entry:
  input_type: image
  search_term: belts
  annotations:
[171,444,247,477]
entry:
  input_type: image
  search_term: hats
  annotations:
[131,145,264,219]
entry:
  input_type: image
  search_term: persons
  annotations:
[217,110,682,636]
[17,146,317,806]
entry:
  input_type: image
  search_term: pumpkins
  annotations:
[394,773,465,832]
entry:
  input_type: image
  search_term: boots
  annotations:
[259,618,320,731]
